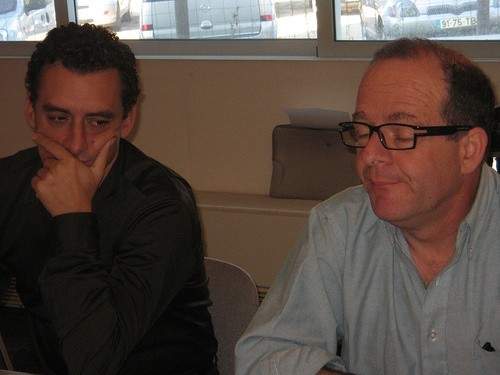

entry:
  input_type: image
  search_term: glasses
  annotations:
[338,119,474,151]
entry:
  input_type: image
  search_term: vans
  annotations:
[139,0,278,39]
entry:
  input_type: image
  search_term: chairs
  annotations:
[203,257,259,375]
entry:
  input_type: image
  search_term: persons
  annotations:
[0,22,221,375]
[234,38,500,375]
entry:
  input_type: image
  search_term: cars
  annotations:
[0,0,56,40]
[357,0,500,40]
[75,0,133,31]
